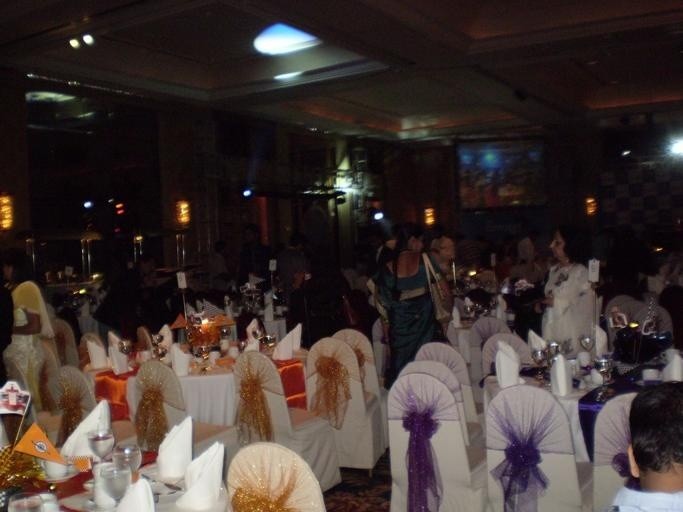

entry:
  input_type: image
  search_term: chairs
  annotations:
[0,268,683,512]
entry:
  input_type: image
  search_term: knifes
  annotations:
[142,473,184,493]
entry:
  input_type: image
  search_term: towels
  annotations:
[663,354,683,382]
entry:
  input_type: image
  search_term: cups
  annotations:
[641,349,680,383]
[36,448,78,475]
[8,492,58,510]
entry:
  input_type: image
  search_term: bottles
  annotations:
[182,271,290,321]
[119,325,282,367]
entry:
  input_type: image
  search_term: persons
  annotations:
[609,382,683,512]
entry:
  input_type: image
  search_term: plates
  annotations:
[33,474,78,484]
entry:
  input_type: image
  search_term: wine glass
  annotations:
[436,265,518,323]
[43,267,88,284]
[532,335,620,400]
[86,425,144,511]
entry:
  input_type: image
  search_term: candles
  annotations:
[588,257,600,283]
[269,258,276,271]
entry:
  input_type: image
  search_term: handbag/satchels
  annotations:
[419,250,455,326]
